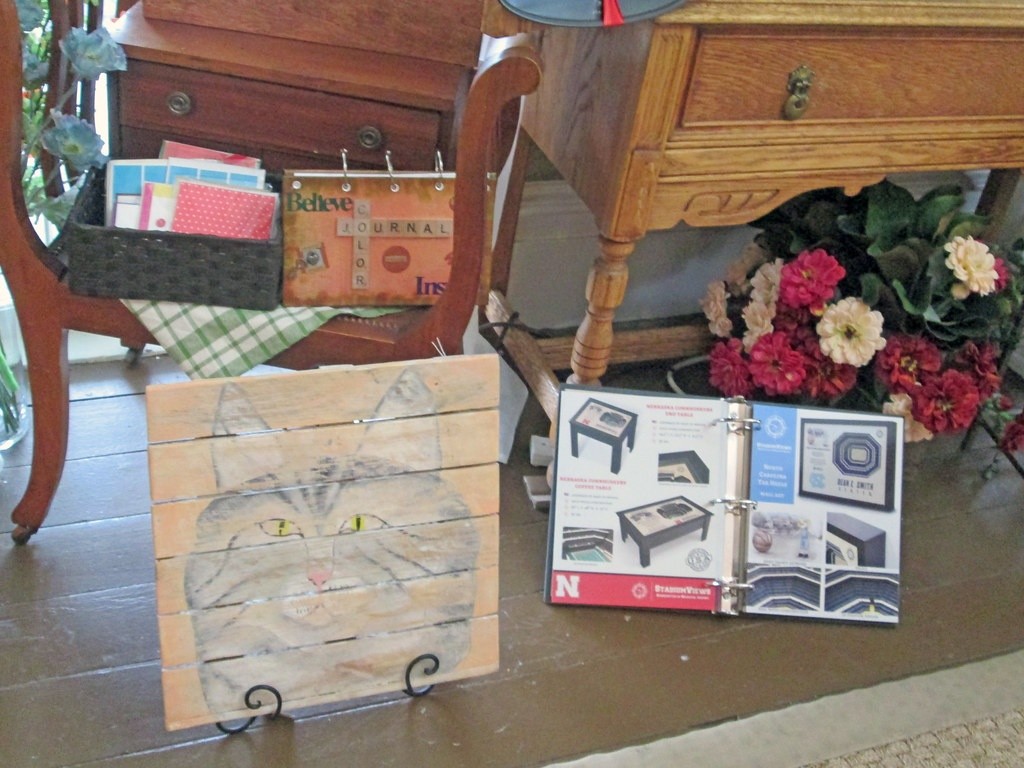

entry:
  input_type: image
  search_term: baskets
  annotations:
[67,166,285,311]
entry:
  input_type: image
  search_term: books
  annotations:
[545,382,902,628]
[278,151,496,307]
[100,138,279,241]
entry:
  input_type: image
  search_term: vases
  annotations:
[0,1,127,434]
[0,275,30,452]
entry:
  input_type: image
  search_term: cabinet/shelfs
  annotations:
[479,0,1024,495]
[108,0,490,174]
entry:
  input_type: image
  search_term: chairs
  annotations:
[0,0,542,548]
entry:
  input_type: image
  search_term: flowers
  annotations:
[703,176,1024,482]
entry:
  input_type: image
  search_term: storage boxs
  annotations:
[66,162,283,311]
